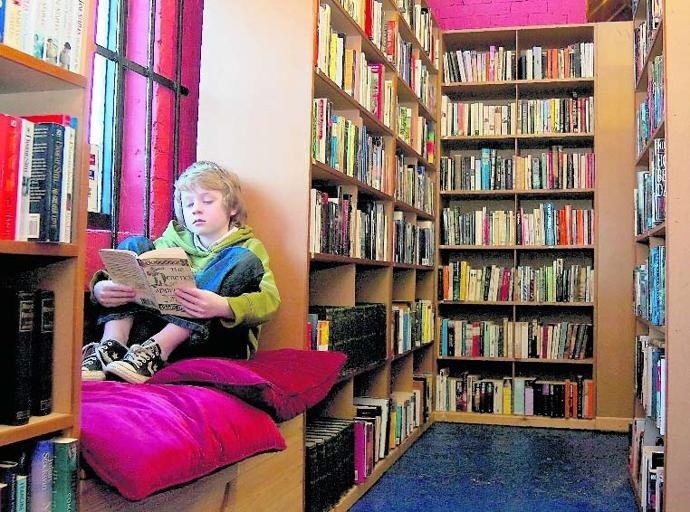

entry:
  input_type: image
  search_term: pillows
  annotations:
[80,377,287,501]
[145,347,347,424]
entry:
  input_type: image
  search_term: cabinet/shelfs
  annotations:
[631,2,690,512]
[0,0,98,512]
[441,22,595,426]
[200,0,438,511]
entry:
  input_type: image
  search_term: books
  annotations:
[308,300,435,355]
[395,154,434,214]
[518,42,594,80]
[310,189,388,261]
[437,317,593,360]
[97,247,204,320]
[1,112,77,243]
[438,260,594,303]
[307,374,434,511]
[627,0,666,512]
[313,96,385,192]
[440,203,594,245]
[517,91,594,135]
[440,149,515,191]
[441,96,515,136]
[443,46,516,84]
[517,146,594,190]
[436,367,593,419]
[318,0,438,165]
[1,438,79,512]
[1,286,55,425]
[394,211,433,266]
[0,1,84,73]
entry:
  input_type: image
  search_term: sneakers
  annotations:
[82,337,167,384]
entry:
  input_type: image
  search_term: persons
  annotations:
[81,160,282,386]
